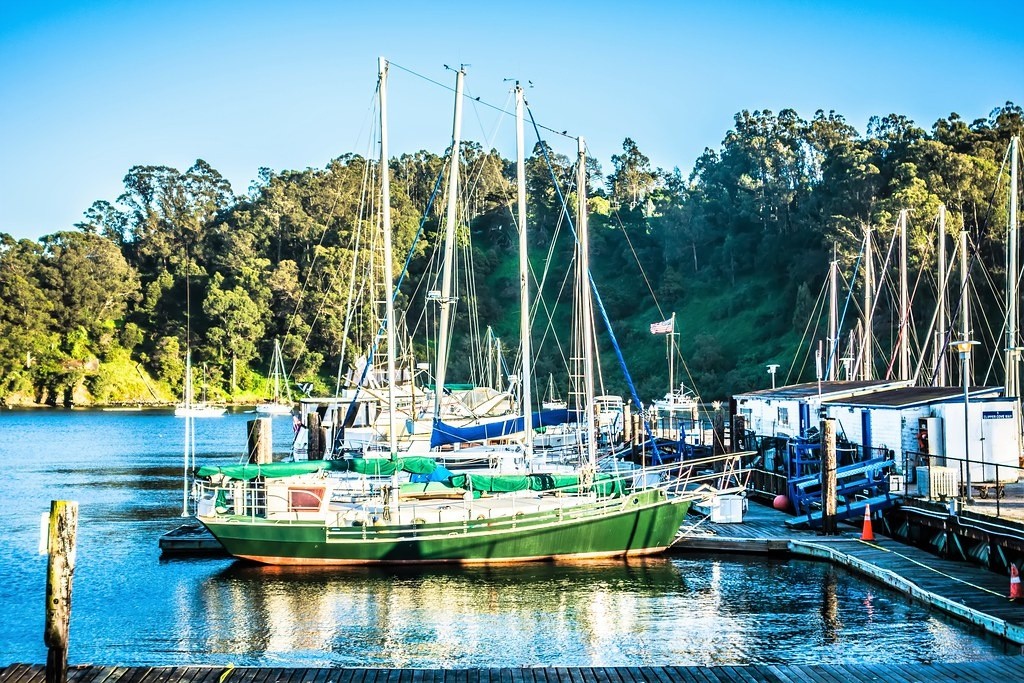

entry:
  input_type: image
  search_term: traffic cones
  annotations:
[859,504,877,540]
[1007,563,1024,599]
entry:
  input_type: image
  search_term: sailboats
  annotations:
[154,50,1024,576]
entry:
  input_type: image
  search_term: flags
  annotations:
[650,318,673,334]
